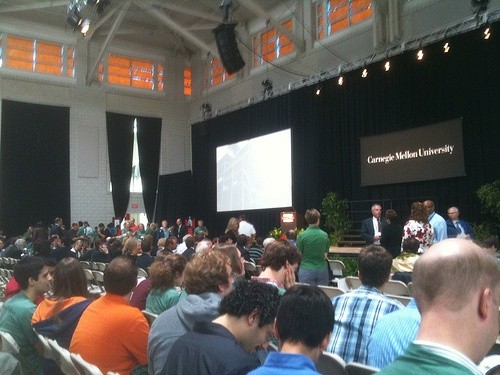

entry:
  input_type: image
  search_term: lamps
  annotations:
[67,7,90,36]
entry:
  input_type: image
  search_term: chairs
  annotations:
[244,257,500,375]
[0,258,158,375]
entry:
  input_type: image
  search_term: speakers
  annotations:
[214,23,245,75]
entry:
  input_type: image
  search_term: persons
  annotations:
[32,254,92,351]
[0,255,54,375]
[367,282,423,372]
[147,248,234,375]
[373,237,500,375]
[296,209,330,287]
[401,201,437,256]
[423,200,447,244]
[392,237,420,284]
[249,238,301,349]
[481,239,498,255]
[361,203,387,246]
[161,282,282,375]
[381,210,403,260]
[324,244,404,369]
[446,206,476,240]
[247,285,334,375]
[69,256,151,375]
[146,253,188,317]
[5,215,296,279]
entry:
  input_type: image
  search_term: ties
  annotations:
[455,222,462,234]
[377,219,381,237]
